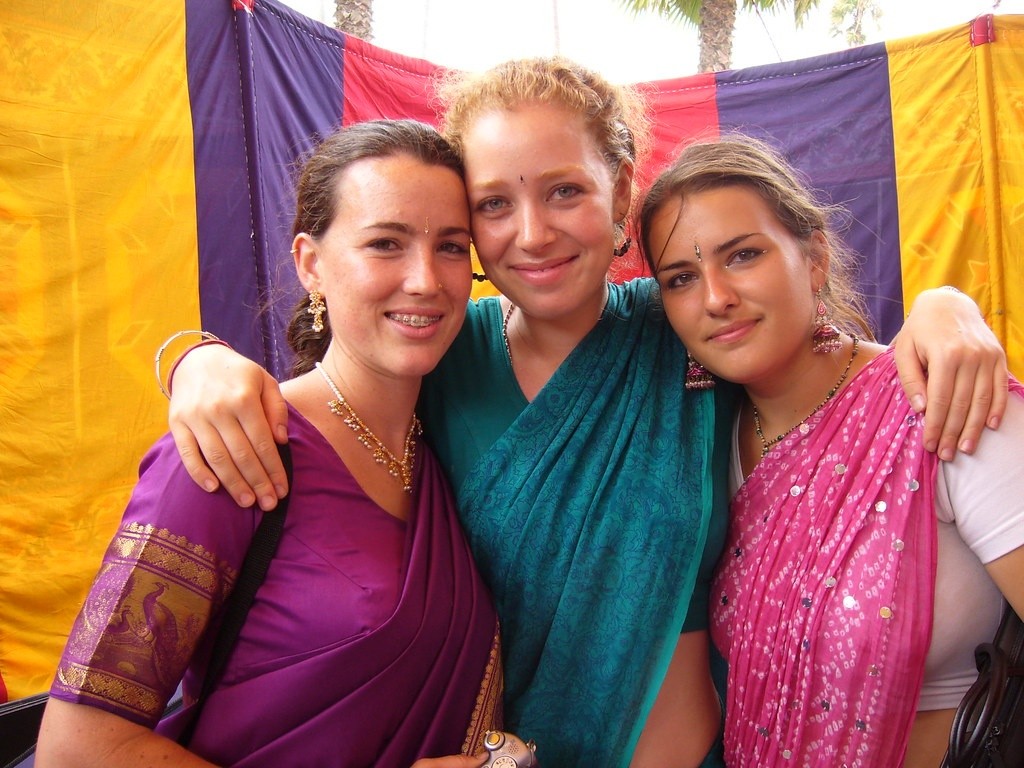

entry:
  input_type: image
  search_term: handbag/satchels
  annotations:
[937,599,1024,768]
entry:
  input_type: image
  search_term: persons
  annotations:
[634,129,1024,767]
[157,60,1009,768]
[29,119,504,768]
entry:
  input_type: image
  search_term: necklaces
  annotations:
[315,361,423,494]
[754,332,858,459]
[504,304,516,368]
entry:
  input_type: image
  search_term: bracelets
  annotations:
[167,339,235,394]
[156,330,221,403]
